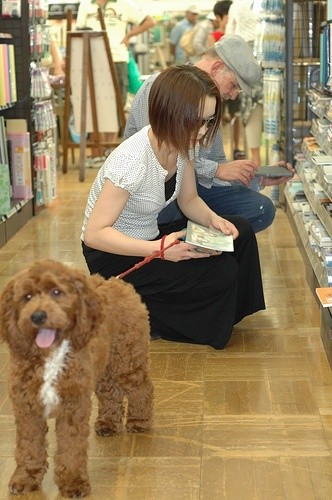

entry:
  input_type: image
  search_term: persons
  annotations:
[166,0,264,165]
[123,33,296,233]
[75,0,156,157]
[80,64,266,350]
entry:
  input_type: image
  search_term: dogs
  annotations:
[1,258,155,498]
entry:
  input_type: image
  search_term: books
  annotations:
[316,287,332,307]
[254,166,293,178]
[185,220,234,253]
[284,136,332,287]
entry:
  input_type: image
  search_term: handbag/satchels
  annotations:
[126,48,141,95]
[180,20,213,56]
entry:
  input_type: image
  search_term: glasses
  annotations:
[183,114,218,129]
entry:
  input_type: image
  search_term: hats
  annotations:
[185,5,200,13]
[214,34,264,95]
